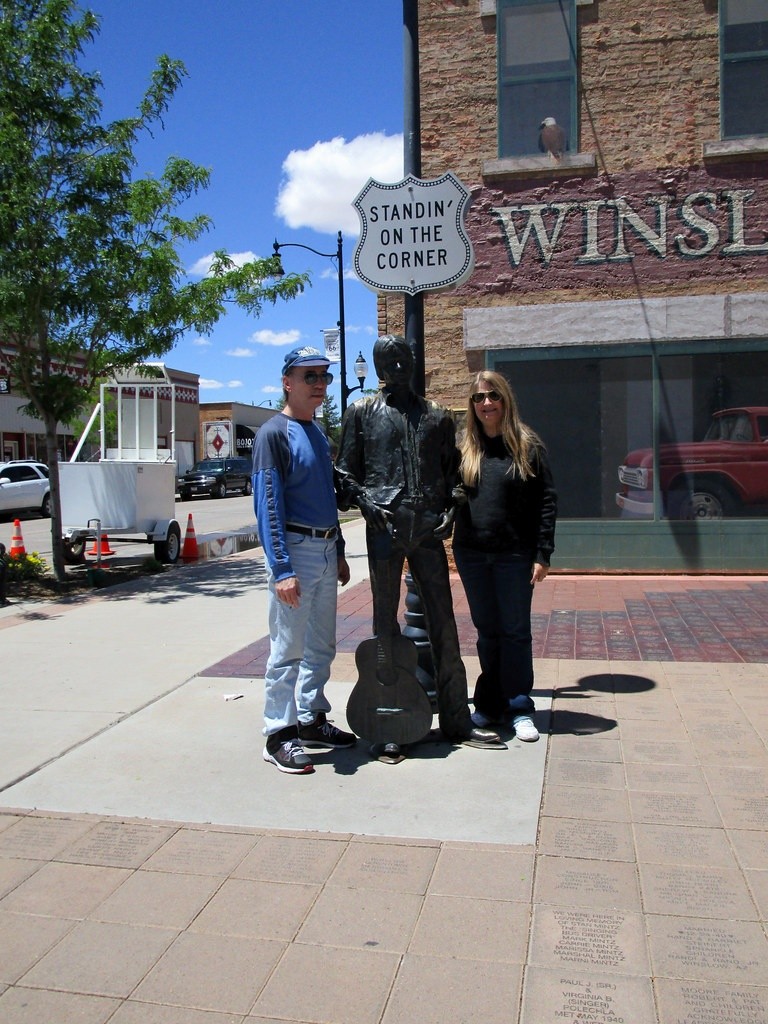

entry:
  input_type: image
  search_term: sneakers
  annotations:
[471,710,498,727]
[298,713,360,749]
[512,712,539,742]
[263,727,311,774]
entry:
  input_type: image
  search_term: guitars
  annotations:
[346,510,434,748]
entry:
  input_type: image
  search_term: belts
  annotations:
[286,522,338,539]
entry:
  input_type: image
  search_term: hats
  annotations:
[282,346,338,374]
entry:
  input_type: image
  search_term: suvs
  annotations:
[0,458,51,518]
[179,456,253,501]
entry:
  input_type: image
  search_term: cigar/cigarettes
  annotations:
[290,602,294,609]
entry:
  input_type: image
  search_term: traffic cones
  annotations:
[86,534,116,556]
[10,519,25,556]
[178,513,201,559]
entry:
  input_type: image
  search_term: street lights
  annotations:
[272,229,369,418]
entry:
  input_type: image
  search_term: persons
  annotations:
[333,333,501,754]
[450,370,558,742]
[251,345,357,775]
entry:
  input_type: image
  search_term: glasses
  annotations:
[471,390,500,404]
[294,371,333,385]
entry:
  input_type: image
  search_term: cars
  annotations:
[614,405,768,520]
